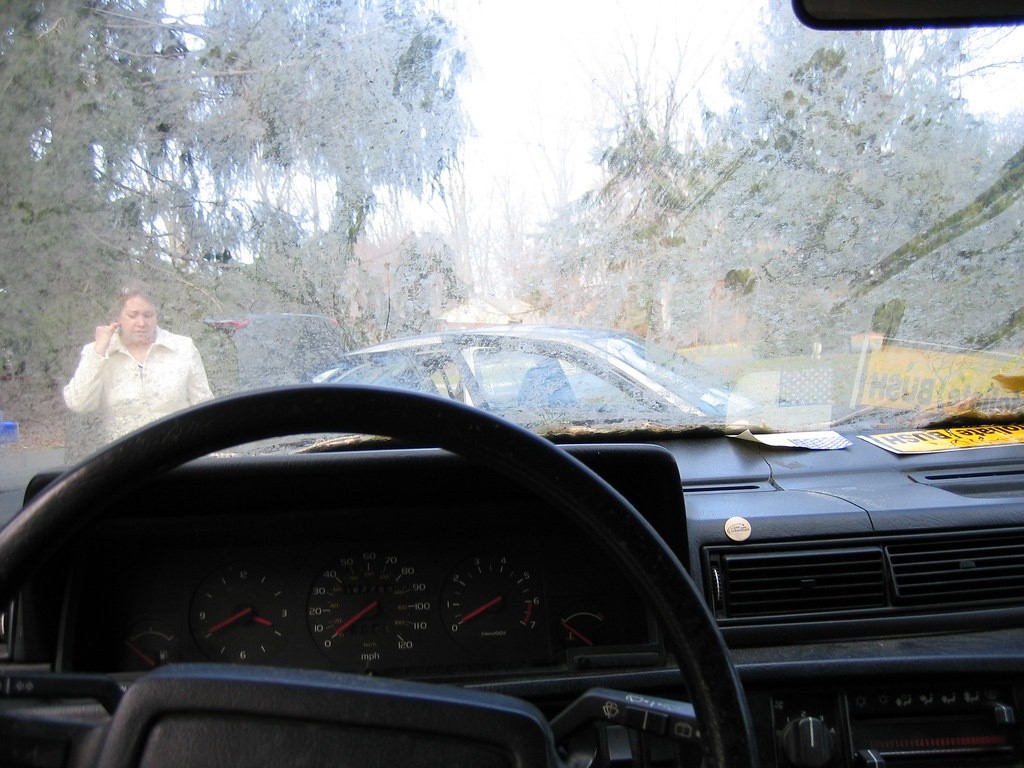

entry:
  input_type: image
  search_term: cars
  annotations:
[263,322,763,431]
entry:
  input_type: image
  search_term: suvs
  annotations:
[184,311,363,400]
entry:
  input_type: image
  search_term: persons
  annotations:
[61,291,217,464]
